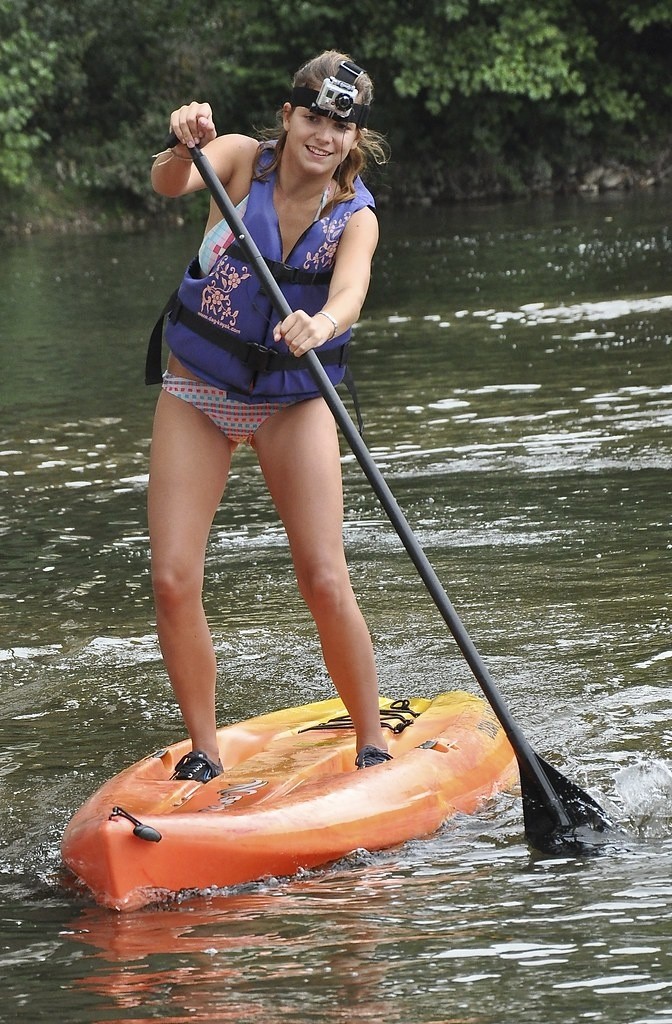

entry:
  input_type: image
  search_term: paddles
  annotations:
[157,94,609,853]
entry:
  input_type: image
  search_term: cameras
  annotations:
[316,76,358,117]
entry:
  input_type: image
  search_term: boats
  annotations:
[61,690,520,913]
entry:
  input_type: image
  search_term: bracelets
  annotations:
[314,312,338,342]
[151,146,193,165]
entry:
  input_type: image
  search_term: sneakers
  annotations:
[169,749,225,785]
[354,744,393,771]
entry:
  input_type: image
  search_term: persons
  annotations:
[147,49,394,788]
